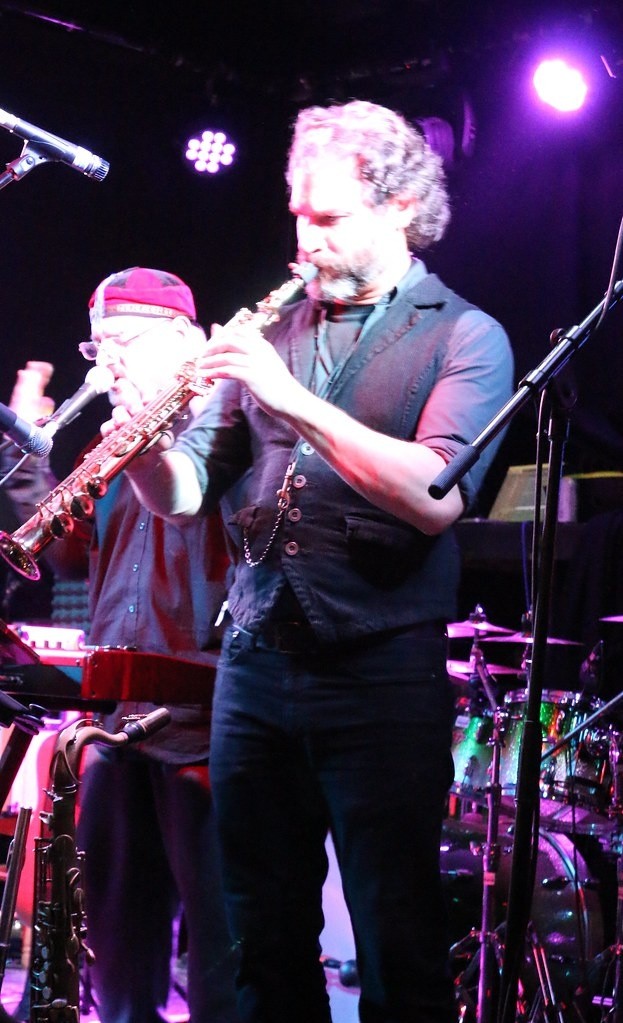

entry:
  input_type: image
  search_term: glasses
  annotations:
[78,318,171,361]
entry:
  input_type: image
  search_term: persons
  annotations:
[1,269,231,1022]
[102,104,514,1023]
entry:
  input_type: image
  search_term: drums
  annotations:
[448,695,493,809]
[440,811,606,999]
[483,687,618,835]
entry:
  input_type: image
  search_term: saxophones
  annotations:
[28,705,173,1023]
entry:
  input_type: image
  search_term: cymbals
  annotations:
[445,602,623,682]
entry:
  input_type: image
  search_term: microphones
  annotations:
[0,108,111,182]
[579,640,604,680]
[42,366,115,438]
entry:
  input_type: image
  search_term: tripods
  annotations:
[447,605,528,1023]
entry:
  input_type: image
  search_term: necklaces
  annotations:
[277,309,358,511]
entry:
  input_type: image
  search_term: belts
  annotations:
[227,619,446,655]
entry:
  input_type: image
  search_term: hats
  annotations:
[87,266,197,321]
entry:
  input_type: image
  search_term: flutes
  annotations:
[0,257,320,582]
